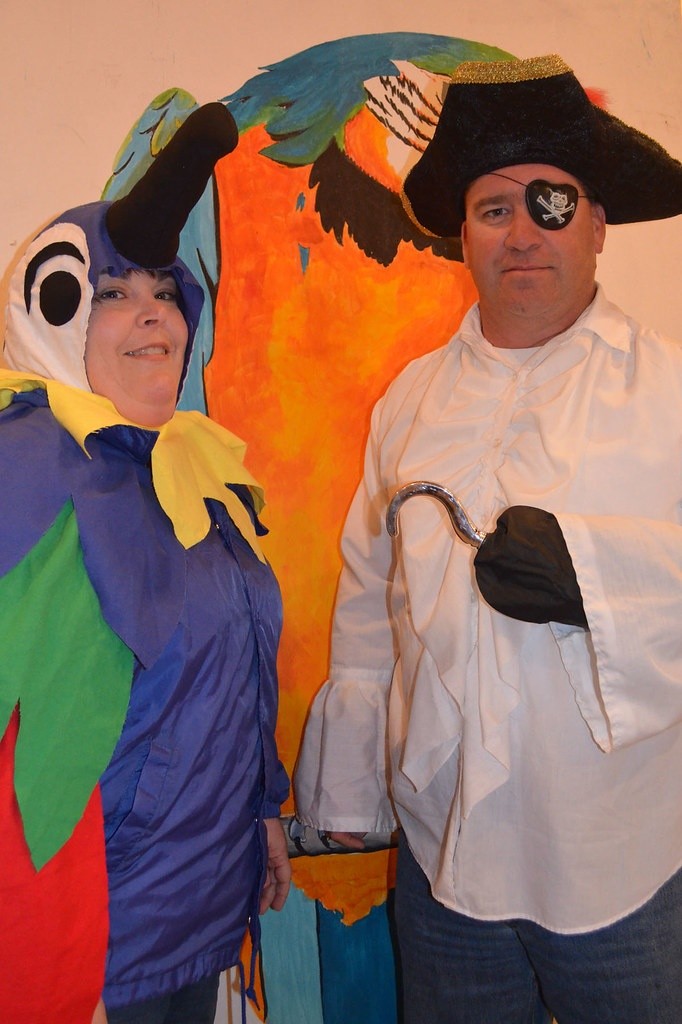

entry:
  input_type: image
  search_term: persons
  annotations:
[294,57,681,1021]
[0,104,291,1024]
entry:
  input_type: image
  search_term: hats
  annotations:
[403,55,682,240]
